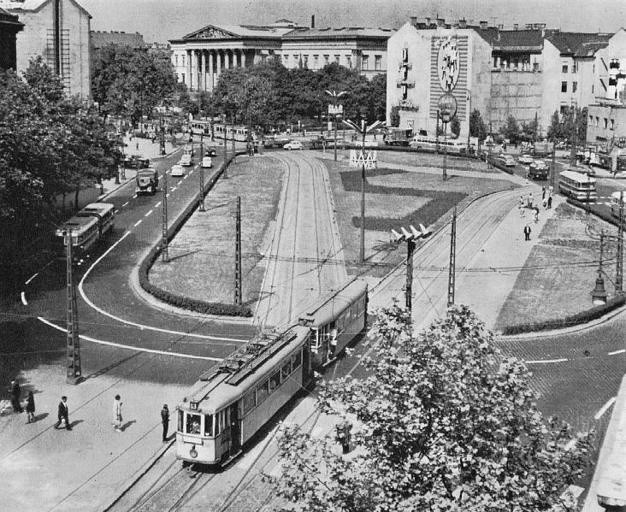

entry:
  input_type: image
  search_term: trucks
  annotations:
[135,168,159,196]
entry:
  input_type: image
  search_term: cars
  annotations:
[169,165,184,177]
[205,146,216,157]
[202,157,212,168]
[529,161,549,177]
[189,120,557,168]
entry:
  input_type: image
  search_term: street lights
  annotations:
[334,114,386,265]
[391,223,432,330]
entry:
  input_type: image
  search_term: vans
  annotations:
[180,154,193,166]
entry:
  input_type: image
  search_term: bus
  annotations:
[558,170,597,202]
[610,191,626,221]
[51,203,113,272]
[175,274,367,469]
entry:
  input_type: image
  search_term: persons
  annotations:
[328,328,338,360]
[24,390,36,425]
[160,403,171,444]
[248,145,255,156]
[501,140,507,152]
[517,183,554,242]
[6,377,24,414]
[54,396,73,431]
[372,133,378,143]
[112,394,124,434]
